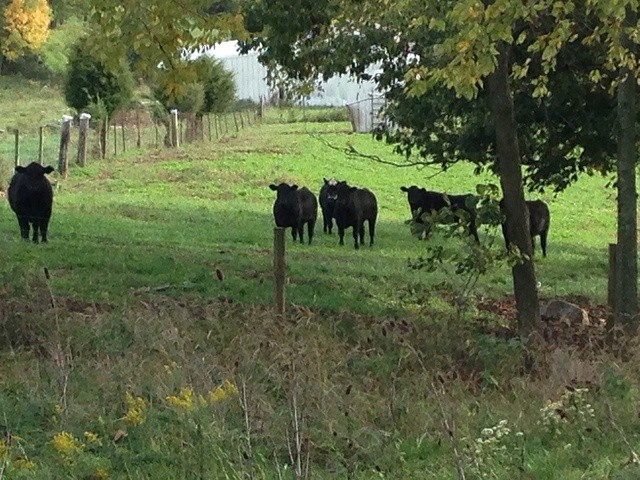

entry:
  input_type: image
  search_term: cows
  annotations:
[495,199,551,258]
[399,185,479,245]
[324,177,378,251]
[7,161,55,246]
[320,176,346,235]
[268,182,318,248]
